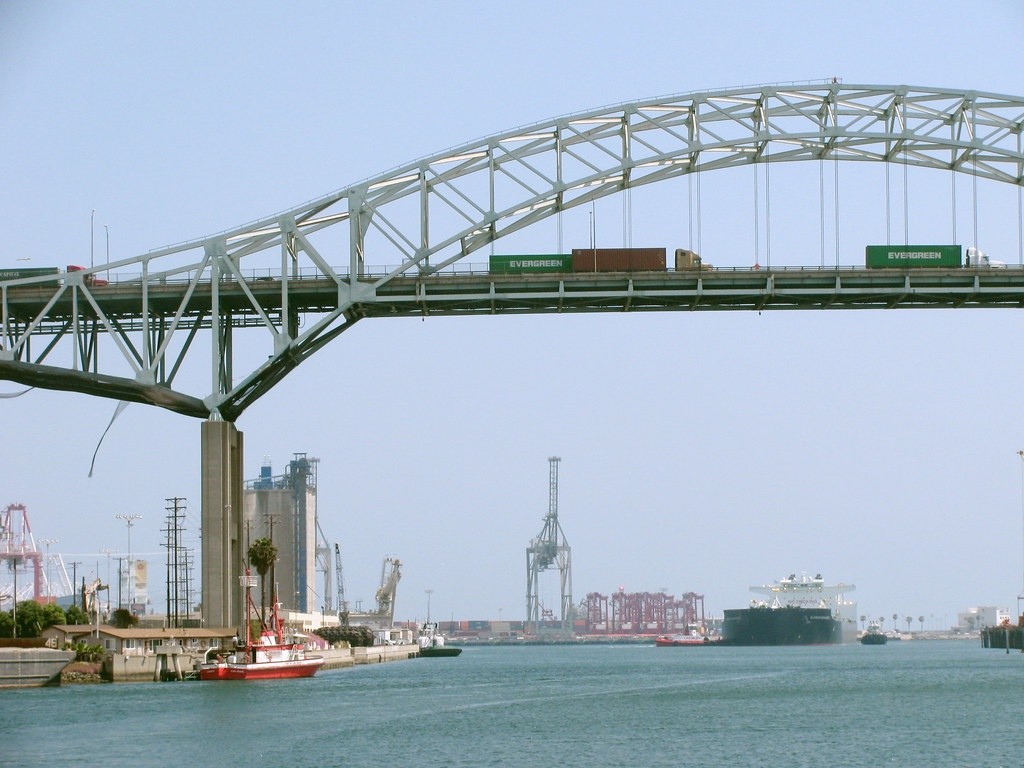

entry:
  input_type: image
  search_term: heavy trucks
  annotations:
[0,264,97,288]
[487,247,703,271]
[865,244,989,269]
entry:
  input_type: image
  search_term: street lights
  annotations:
[100,548,114,587]
[90,209,96,272]
[116,513,143,612]
[104,224,110,283]
[590,211,593,249]
[38,539,59,607]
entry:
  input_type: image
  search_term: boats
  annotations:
[655,630,712,647]
[859,621,888,645]
[198,568,325,681]
